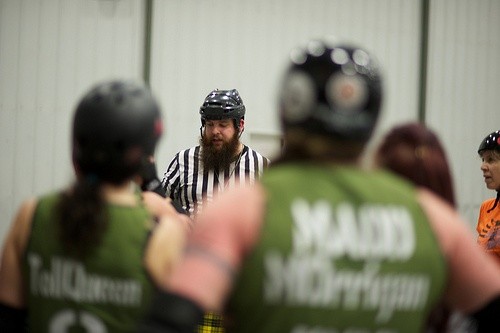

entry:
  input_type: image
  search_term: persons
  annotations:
[473,129,500,333]
[163,89,272,220]
[371,124,458,333]
[0,77,206,332]
[146,37,499,332]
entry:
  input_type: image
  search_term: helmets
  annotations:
[73,80,164,180]
[279,42,386,141]
[478,130,500,153]
[199,89,245,121]
